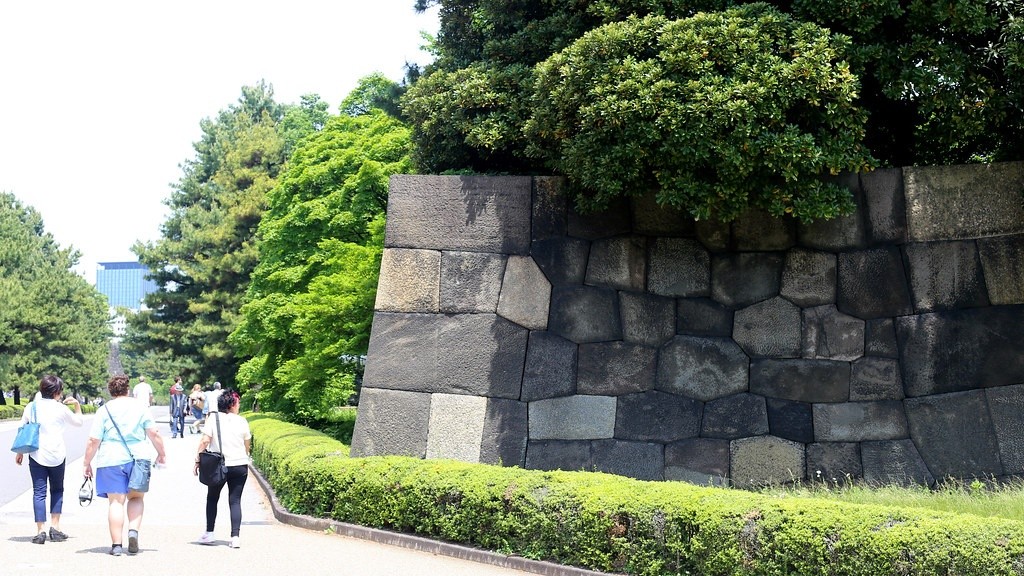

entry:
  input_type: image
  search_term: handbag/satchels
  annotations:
[10,401,41,454]
[192,399,203,411]
[77,476,93,506]
[127,458,152,492]
[198,449,227,488]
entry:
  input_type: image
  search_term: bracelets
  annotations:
[195,459,200,464]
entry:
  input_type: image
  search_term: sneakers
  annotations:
[127,529,138,553]
[32,532,46,544]
[49,527,68,541]
[228,536,241,548]
[190,533,215,545]
[109,544,123,556]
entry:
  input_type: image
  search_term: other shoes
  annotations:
[171,433,177,438]
[196,430,203,434]
[189,424,194,434]
[180,433,184,438]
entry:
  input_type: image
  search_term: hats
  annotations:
[174,385,184,392]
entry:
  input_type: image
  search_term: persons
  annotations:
[193,391,251,548]
[203,385,211,418]
[133,376,153,408]
[189,384,205,434]
[83,375,166,556]
[208,382,225,412]
[170,376,182,432]
[15,376,83,544]
[170,385,187,438]
